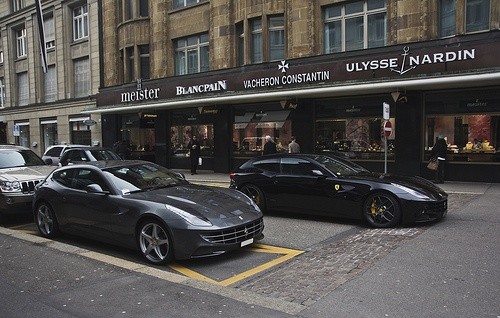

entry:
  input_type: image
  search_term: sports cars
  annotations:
[230,150,448,229]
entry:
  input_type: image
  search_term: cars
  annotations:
[60,144,121,168]
[32,161,266,264]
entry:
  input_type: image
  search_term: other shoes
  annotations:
[191,173,194,175]
[194,173,197,174]
[434,182,439,184]
[440,182,444,184]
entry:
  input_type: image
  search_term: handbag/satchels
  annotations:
[426,161,438,170]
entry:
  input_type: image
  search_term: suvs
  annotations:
[239,138,287,155]
[39,145,96,163]
[0,144,52,225]
[334,137,395,161]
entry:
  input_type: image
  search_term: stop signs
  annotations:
[383,118,393,139]
